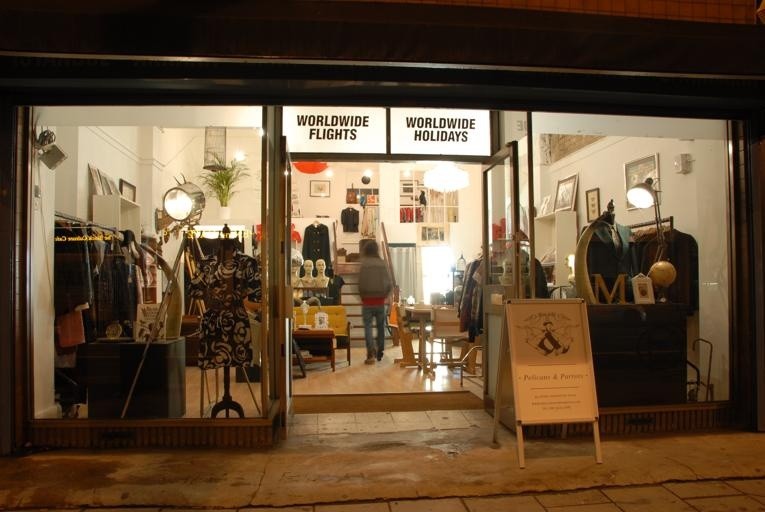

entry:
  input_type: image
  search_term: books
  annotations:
[292,349,312,359]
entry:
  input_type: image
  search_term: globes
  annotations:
[649,261,677,304]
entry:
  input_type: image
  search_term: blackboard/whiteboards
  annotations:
[505,298,600,425]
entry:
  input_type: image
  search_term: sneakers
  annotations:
[364,352,384,365]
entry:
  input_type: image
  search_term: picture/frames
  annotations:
[586,188,602,222]
[553,173,578,212]
[309,180,330,198]
[632,277,656,304]
[314,312,328,329]
[120,177,136,202]
[623,152,662,211]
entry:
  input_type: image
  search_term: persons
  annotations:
[581,212,633,303]
[357,239,393,365]
[186,238,262,371]
[290,248,303,288]
[315,258,330,288]
[300,259,316,288]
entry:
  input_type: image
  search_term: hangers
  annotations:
[628,220,676,243]
[52,209,124,246]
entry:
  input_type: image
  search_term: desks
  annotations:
[291,329,336,371]
[391,302,479,380]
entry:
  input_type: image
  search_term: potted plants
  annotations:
[196,150,250,223]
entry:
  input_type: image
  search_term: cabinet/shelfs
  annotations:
[92,195,141,245]
[536,211,578,289]
[75,336,185,420]
[585,302,689,408]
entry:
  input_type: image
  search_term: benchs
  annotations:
[291,305,352,366]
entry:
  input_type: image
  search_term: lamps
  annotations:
[163,181,206,232]
[424,163,470,193]
[626,176,668,303]
